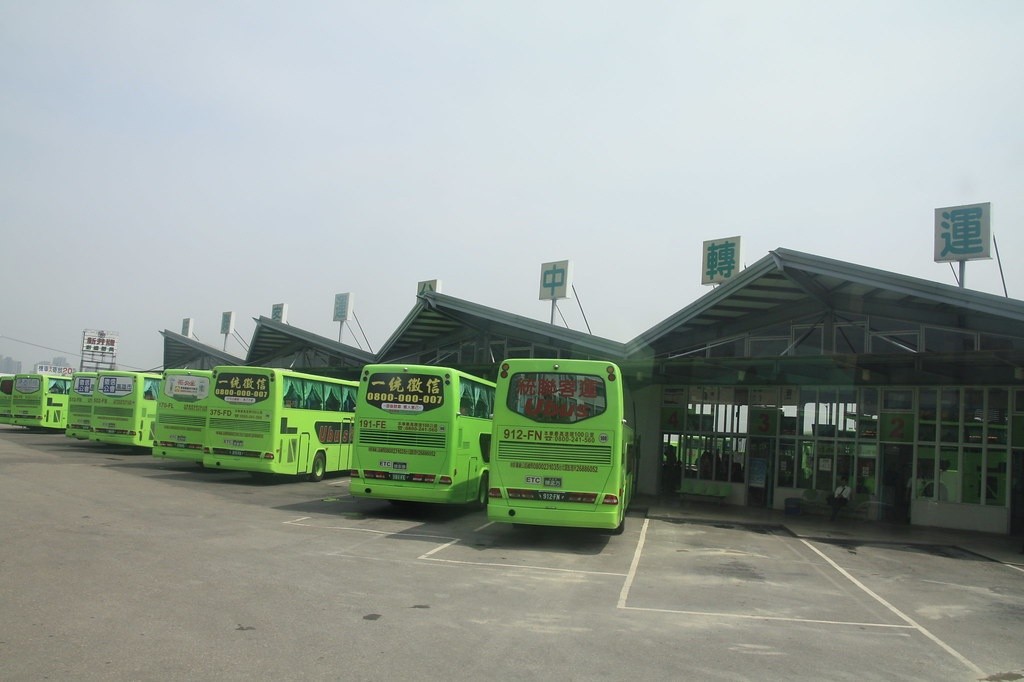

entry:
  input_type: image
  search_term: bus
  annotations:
[202,366,360,482]
[152,370,212,466]
[486,359,638,535]
[88,370,163,448]
[65,371,97,440]
[0,376,15,424]
[10,373,73,430]
[347,363,496,510]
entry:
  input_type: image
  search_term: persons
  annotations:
[831,477,851,521]
[664,444,744,480]
[884,463,899,491]
[906,468,923,522]
